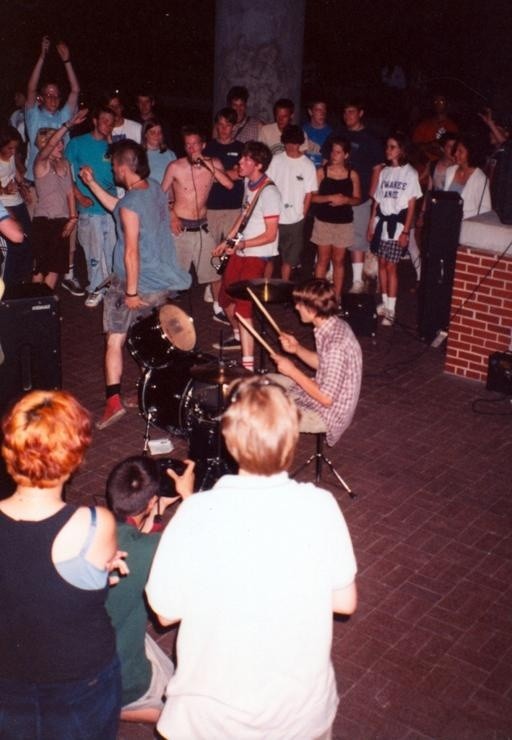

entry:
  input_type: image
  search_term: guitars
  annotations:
[215,232,244,276]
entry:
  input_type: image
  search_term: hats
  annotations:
[279,124,305,145]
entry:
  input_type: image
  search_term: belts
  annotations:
[182,222,209,234]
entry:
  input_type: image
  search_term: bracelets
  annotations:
[241,240,249,256]
[66,215,80,221]
[124,292,138,298]
[85,178,96,186]
[61,119,75,132]
[39,53,46,61]
[401,227,412,236]
[60,58,73,66]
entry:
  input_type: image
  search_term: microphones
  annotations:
[197,158,211,172]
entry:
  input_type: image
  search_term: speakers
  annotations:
[0,283,63,410]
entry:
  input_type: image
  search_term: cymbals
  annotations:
[225,278,303,305]
[189,360,254,383]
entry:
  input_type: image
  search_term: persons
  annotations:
[107,93,144,147]
[64,106,118,310]
[23,34,82,186]
[411,94,461,218]
[208,139,283,377]
[140,117,178,210]
[428,127,460,189]
[9,87,28,144]
[442,135,492,220]
[476,102,512,223]
[224,84,266,143]
[268,123,319,284]
[102,451,198,727]
[368,134,423,296]
[311,134,363,313]
[31,107,89,300]
[0,124,33,297]
[260,96,311,160]
[133,87,162,126]
[262,277,366,449]
[141,371,364,740]
[75,138,195,434]
[366,130,425,328]
[0,385,135,740]
[305,97,332,178]
[0,200,27,280]
[323,101,374,299]
[160,128,233,328]
[204,106,246,304]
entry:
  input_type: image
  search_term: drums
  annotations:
[125,302,199,369]
[178,360,246,446]
[137,351,217,438]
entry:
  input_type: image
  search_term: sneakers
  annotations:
[84,291,102,307]
[62,275,87,297]
[211,338,243,351]
[93,393,127,431]
[381,309,396,327]
[212,311,232,325]
[377,301,385,318]
[126,387,145,410]
[204,284,215,303]
[349,278,365,294]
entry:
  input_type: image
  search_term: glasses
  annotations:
[385,145,396,150]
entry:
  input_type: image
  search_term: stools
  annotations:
[290,434,358,498]
